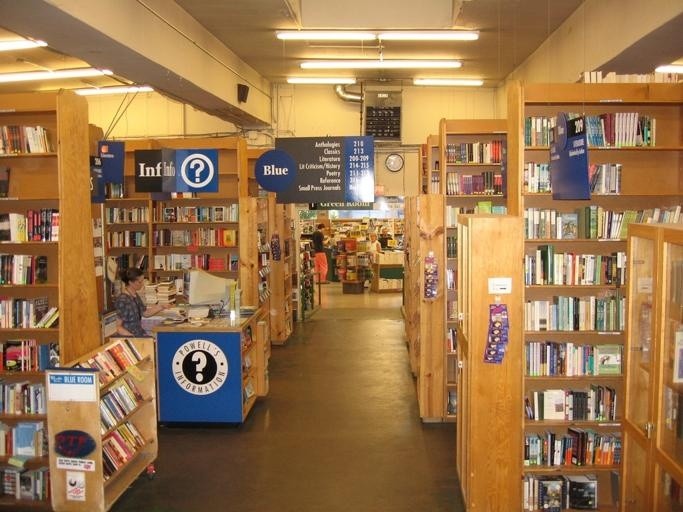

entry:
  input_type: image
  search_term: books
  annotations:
[240,183,314,406]
[328,217,405,290]
[402,70,682,510]
[91,182,240,338]
[1,125,59,502]
[70,338,146,483]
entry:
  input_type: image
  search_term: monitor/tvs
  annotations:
[181,267,236,305]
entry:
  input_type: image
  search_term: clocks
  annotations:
[385,154,404,172]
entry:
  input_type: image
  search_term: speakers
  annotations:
[238,83,249,102]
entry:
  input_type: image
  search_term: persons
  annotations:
[310,223,331,284]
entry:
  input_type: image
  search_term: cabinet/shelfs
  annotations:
[300,218,405,295]
[0,88,160,511]
[87,123,270,429]
[455,79,683,511]
[619,223,683,512]
[247,148,301,346]
[401,119,508,423]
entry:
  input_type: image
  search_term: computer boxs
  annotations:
[184,303,221,318]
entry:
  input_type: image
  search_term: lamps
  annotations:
[413,77,484,87]
[273,29,480,42]
[297,58,464,69]
[285,76,356,84]
[74,83,154,96]
[655,56,683,73]
[0,68,114,83]
